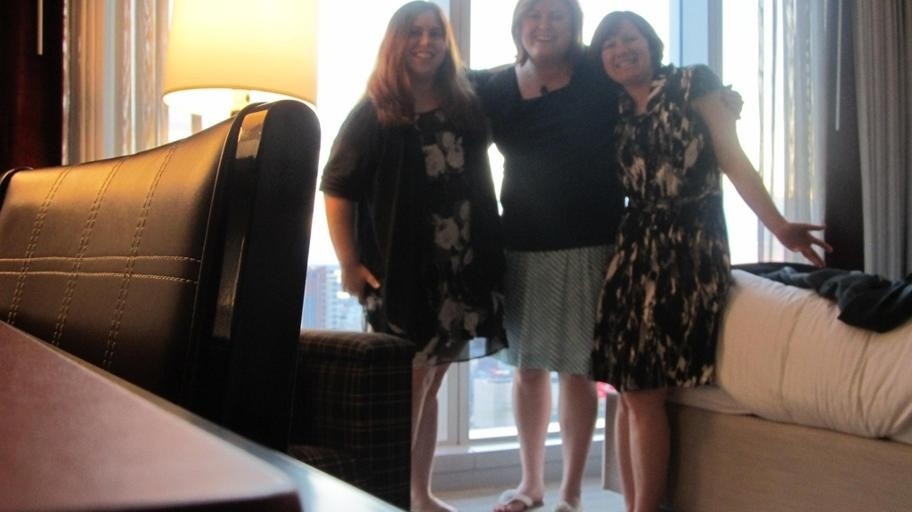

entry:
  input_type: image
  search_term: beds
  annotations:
[600,271,912,512]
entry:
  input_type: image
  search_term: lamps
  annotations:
[163,0,329,122]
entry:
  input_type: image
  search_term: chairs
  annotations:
[296,331,416,509]
[0,99,320,454]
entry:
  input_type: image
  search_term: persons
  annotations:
[316,0,521,511]
[470,0,745,511]
[589,10,838,512]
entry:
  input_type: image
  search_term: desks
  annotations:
[0,322,409,512]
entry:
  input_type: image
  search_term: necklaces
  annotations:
[538,84,552,96]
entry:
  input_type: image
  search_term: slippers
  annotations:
[552,499,583,512]
[491,488,544,512]
